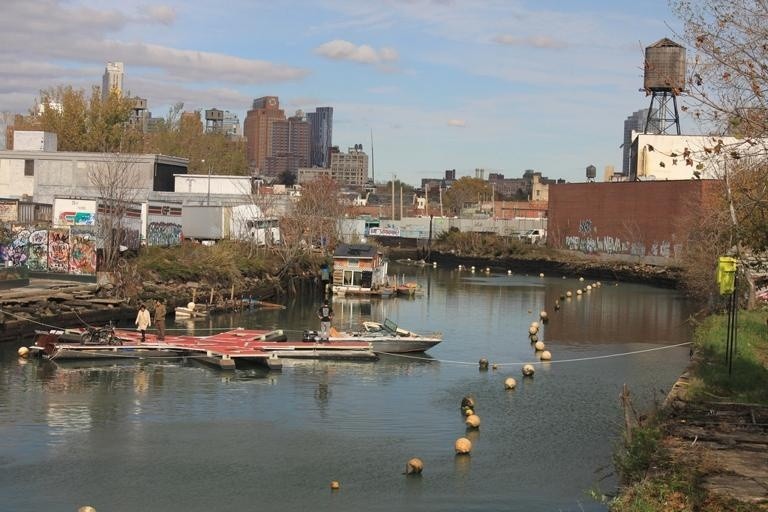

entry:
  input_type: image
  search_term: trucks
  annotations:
[178,203,283,248]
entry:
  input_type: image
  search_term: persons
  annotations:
[134,304,153,341]
[154,297,168,339]
[317,298,335,343]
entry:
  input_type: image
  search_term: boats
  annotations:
[305,320,442,357]
[19,320,183,362]
[174,301,208,319]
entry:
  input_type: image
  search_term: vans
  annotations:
[521,228,547,243]
